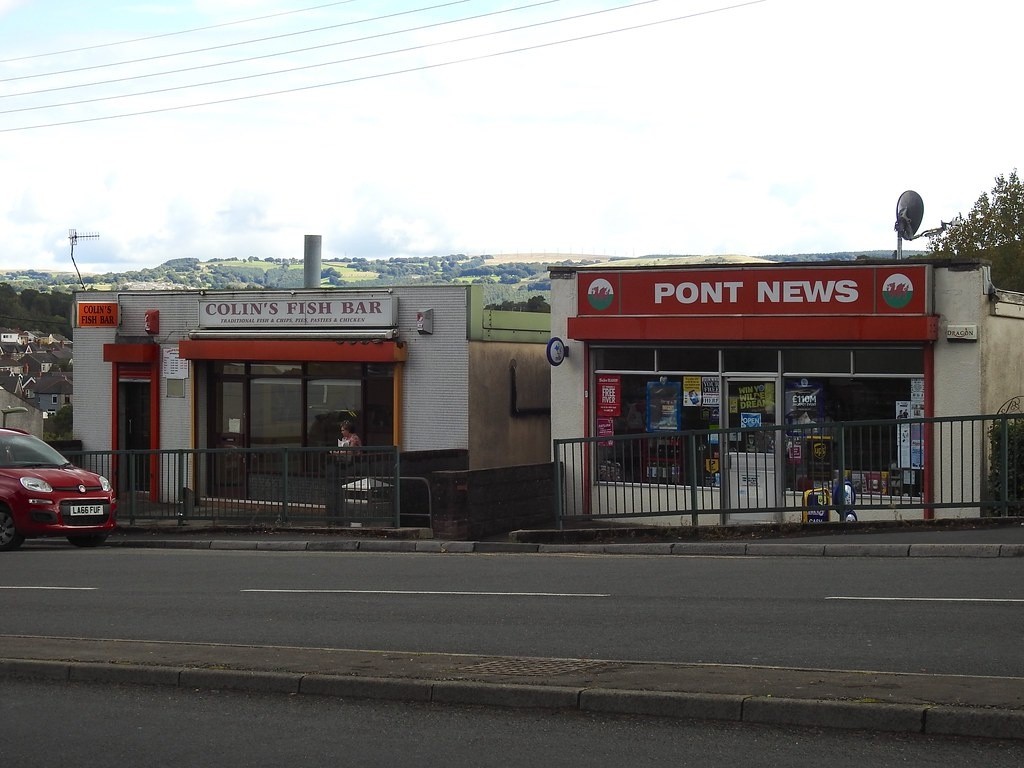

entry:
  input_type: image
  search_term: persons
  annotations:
[333,420,362,477]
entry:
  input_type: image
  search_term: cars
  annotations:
[0,425,118,552]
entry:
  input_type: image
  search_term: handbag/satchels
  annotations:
[337,439,350,447]
